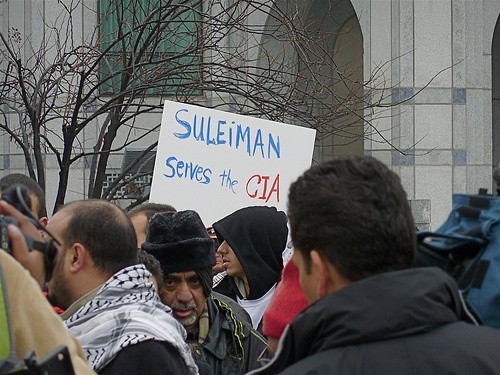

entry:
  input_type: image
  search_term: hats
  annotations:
[206,227,220,249]
[262,253,310,340]
[141,210,216,274]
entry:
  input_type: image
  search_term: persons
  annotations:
[431,162,500,330]
[0,172,198,375]
[257,258,311,368]
[213,206,290,343]
[244,155,500,375]
[204,227,225,277]
[127,203,176,252]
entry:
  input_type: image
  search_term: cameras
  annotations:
[0,215,33,256]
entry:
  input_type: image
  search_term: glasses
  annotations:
[256,346,272,367]
